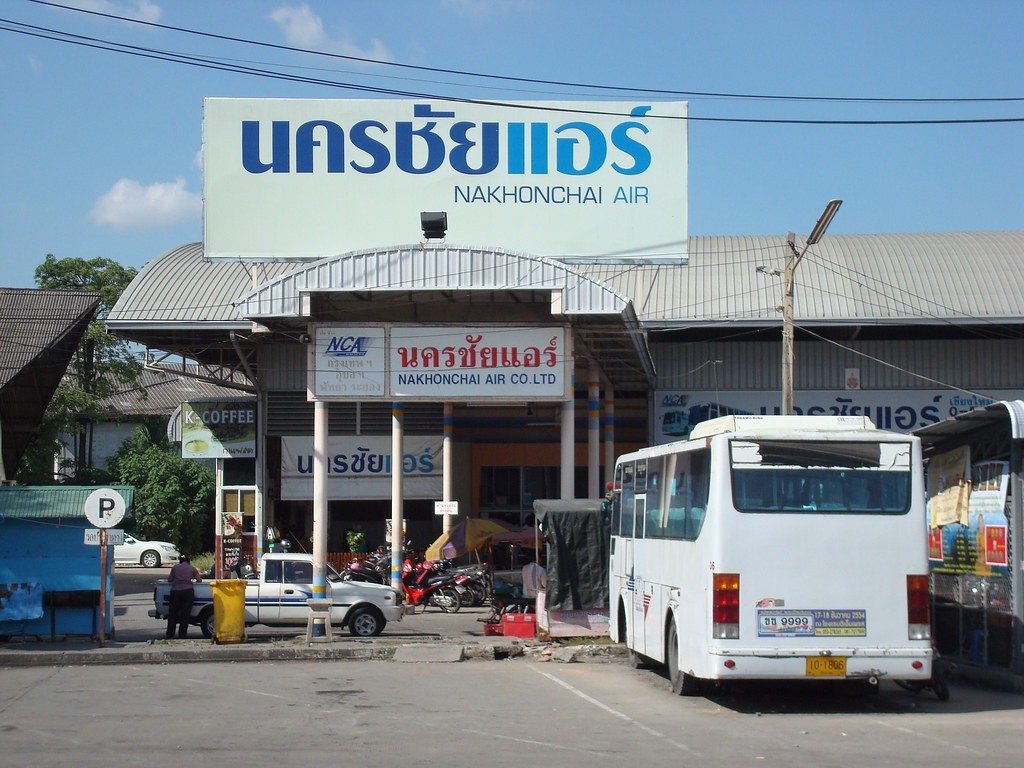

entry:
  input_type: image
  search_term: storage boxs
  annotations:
[501,612,536,638]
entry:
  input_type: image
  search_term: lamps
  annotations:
[420,212,448,243]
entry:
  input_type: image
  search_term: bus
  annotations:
[610,416,933,696]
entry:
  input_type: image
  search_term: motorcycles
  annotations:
[339,551,494,613]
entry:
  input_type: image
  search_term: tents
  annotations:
[533,500,612,611]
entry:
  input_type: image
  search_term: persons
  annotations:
[165,553,202,639]
[521,551,548,605]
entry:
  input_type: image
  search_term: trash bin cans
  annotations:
[209,579,248,644]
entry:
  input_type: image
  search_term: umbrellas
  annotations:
[425,515,543,609]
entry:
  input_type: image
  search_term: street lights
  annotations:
[753,199,844,415]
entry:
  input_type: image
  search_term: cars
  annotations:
[114,532,182,568]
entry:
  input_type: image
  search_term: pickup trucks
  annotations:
[148,553,401,637]
